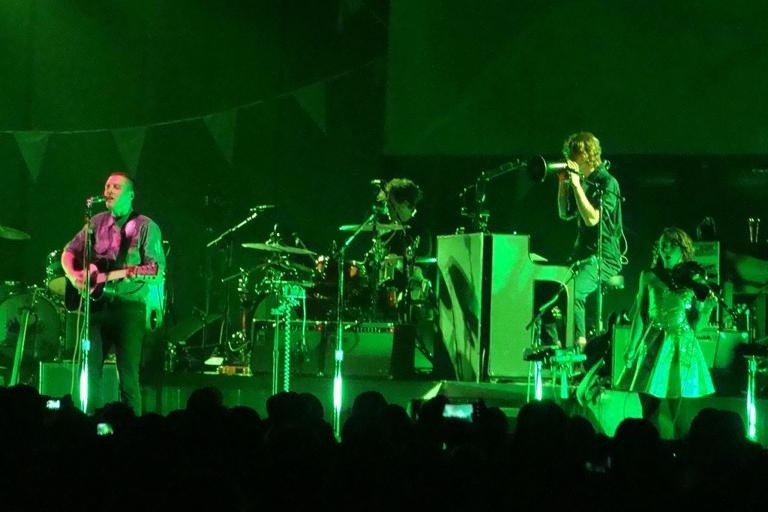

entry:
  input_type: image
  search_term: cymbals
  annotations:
[339,222,412,231]
[1,225,32,241]
[241,243,319,257]
[385,254,437,265]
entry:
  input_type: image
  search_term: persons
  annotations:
[558,132,622,344]
[61,170,159,416]
[617,227,718,439]
[365,177,426,256]
[0,383,768,512]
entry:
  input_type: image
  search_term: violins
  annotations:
[672,261,742,322]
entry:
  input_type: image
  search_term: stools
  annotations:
[589,275,625,337]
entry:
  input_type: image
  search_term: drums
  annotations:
[45,250,66,295]
[242,290,325,353]
[1,285,67,362]
[372,278,400,322]
[3,276,25,291]
[311,256,366,296]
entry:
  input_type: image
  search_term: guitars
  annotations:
[65,258,158,311]
[584,295,638,377]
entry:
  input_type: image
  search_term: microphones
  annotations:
[89,195,107,204]
[249,204,275,211]
[405,235,421,260]
[572,255,597,271]
